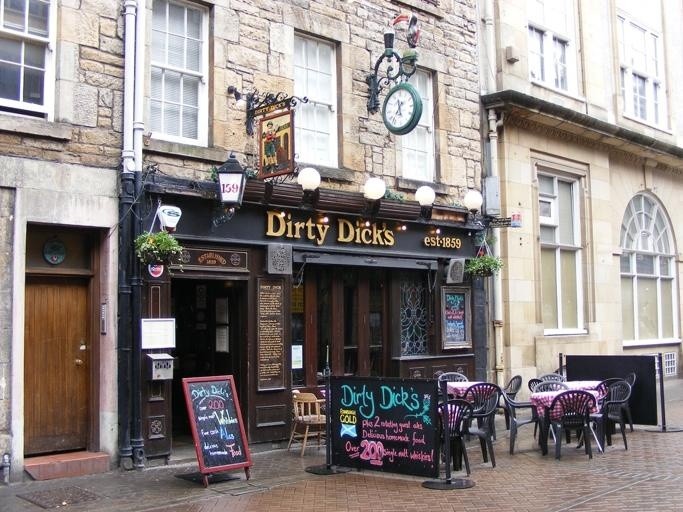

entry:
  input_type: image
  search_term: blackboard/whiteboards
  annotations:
[329,375,440,479]
[182,374,253,474]
[440,286,472,350]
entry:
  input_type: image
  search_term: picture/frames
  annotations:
[258,109,295,178]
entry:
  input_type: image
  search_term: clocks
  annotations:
[381,83,423,135]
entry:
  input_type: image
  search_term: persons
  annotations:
[262,120,279,172]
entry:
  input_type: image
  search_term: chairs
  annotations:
[287,389,332,458]
[436,371,637,476]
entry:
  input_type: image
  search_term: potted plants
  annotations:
[131,229,185,276]
[464,256,504,283]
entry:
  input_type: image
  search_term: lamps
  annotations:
[366,13,420,115]
[362,177,385,214]
[297,167,321,210]
[211,150,249,228]
[464,189,479,222]
[156,204,182,232]
[415,185,435,220]
